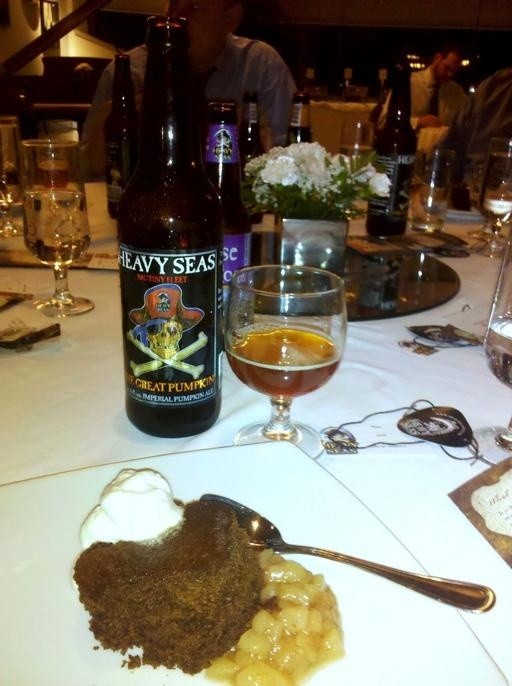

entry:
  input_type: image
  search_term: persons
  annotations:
[432,70,511,183]
[374,42,468,151]
[80,0,295,181]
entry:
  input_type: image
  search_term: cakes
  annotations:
[72,503,267,675]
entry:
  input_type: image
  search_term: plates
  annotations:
[2,437,509,685]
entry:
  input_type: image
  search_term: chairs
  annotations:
[302,81,333,105]
[340,80,369,104]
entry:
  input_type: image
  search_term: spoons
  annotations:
[199,490,499,619]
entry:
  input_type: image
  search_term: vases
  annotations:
[271,212,347,280]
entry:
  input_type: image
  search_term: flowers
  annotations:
[245,140,395,222]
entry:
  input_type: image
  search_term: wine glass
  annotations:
[467,135,511,257]
[336,119,371,218]
[221,263,349,462]
[17,135,98,316]
[0,115,31,240]
[481,229,512,451]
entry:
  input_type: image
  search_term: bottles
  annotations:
[121,14,223,436]
[286,90,313,146]
[235,93,267,228]
[304,60,388,101]
[102,51,141,221]
[204,93,253,351]
[363,61,417,237]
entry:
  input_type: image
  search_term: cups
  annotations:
[35,130,67,187]
[410,145,457,234]
